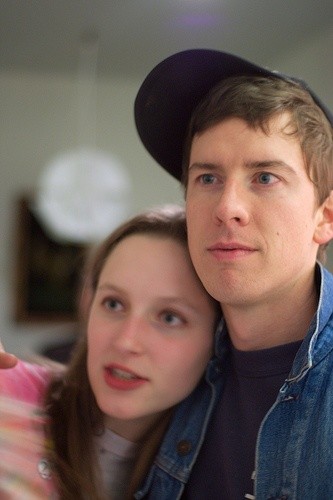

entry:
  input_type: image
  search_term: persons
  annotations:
[0,48,333,500]
[1,207,219,499]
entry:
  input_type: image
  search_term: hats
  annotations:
[132,47,331,183]
